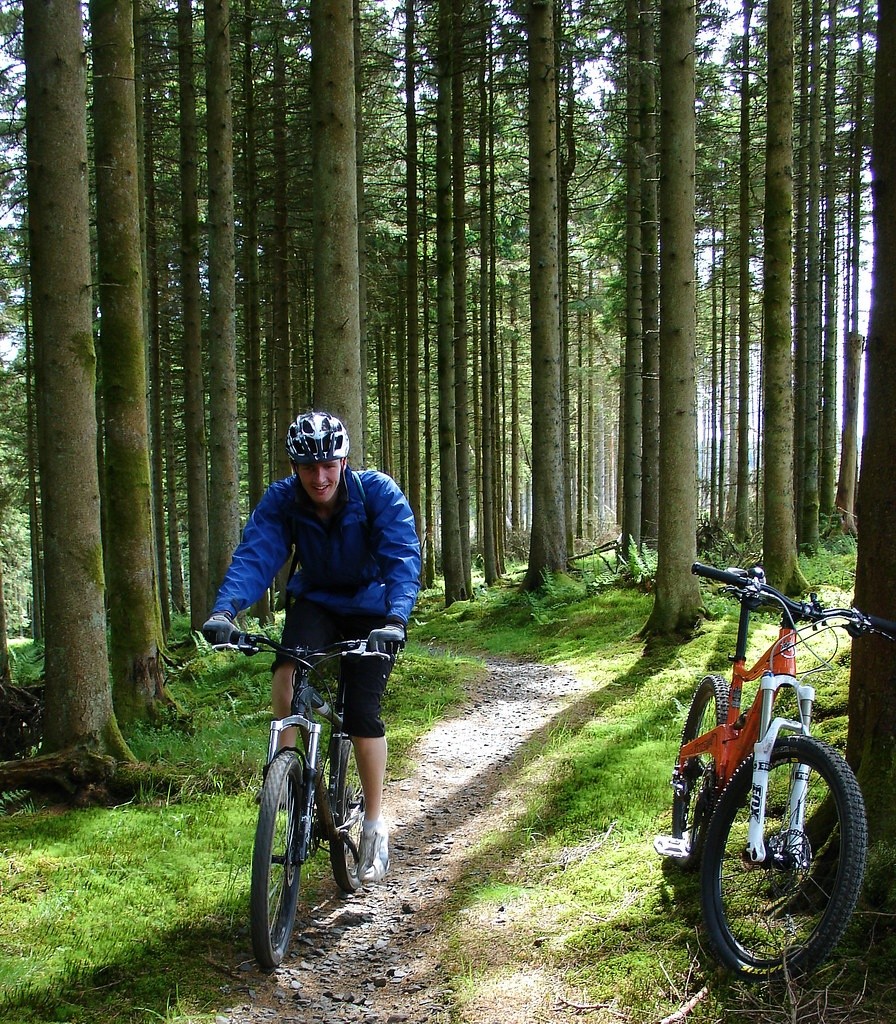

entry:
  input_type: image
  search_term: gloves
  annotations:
[368,623,407,658]
[202,612,239,652]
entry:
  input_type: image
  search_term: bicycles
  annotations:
[210,631,405,971]
[652,561,896,987]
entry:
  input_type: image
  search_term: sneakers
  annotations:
[358,822,389,881]
[253,775,286,809]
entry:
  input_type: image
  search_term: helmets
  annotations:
[285,412,349,465]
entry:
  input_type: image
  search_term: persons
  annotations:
[203,411,422,882]
